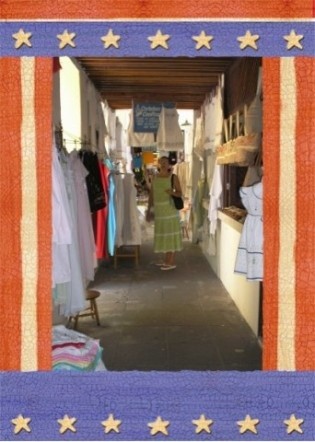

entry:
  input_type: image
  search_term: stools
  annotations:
[114,244,140,269]
[66,290,101,329]
[180,221,189,240]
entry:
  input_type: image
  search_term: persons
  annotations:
[133,151,143,184]
[146,156,184,270]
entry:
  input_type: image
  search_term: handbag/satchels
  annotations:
[171,174,183,210]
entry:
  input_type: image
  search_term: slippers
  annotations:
[150,261,165,266]
[161,264,176,270]
